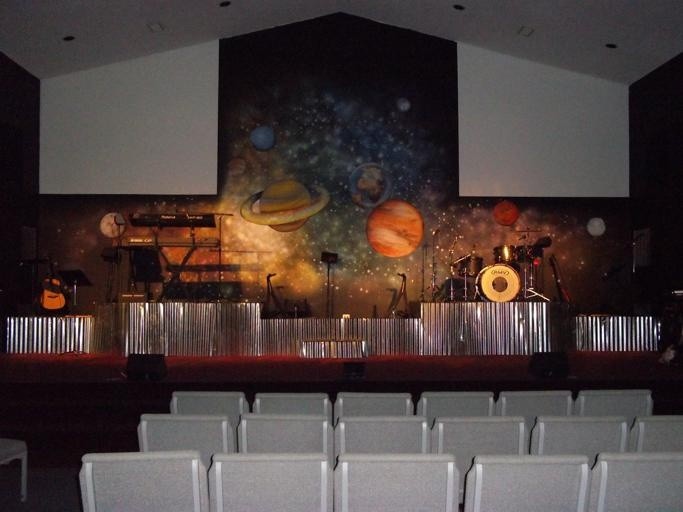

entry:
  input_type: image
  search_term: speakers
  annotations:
[126,354,168,380]
[528,351,570,378]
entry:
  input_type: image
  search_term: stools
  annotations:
[0,439,28,501]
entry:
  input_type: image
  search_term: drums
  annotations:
[475,263,521,303]
[459,254,483,277]
[493,243,516,265]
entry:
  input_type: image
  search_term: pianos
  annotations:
[114,235,219,249]
[127,213,216,227]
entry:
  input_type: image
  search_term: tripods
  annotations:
[441,253,463,302]
[418,233,447,302]
[205,218,241,303]
[458,264,478,302]
[514,240,550,302]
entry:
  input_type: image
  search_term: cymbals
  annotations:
[516,229,540,233]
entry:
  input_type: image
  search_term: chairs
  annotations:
[77,451,212,512]
[464,454,591,512]
[333,454,460,512]
[207,450,334,512]
[137,391,683,454]
[591,451,683,511]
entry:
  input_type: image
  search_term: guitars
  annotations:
[39,258,66,311]
